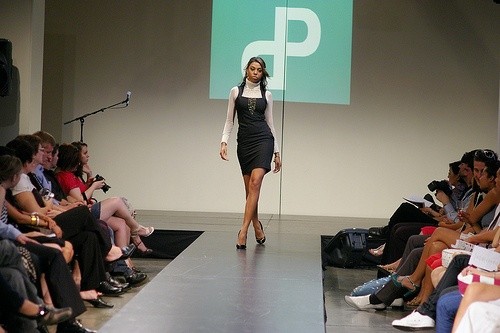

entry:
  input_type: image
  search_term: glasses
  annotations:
[474,150,495,159]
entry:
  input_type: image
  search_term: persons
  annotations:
[344,149,500,333]
[220,57,280,248]
[0,131,155,333]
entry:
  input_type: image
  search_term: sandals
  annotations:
[377,263,396,274]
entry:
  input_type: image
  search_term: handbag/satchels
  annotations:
[457,264,500,297]
[441,247,471,269]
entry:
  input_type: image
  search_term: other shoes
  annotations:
[131,265,142,273]
[390,297,402,308]
[133,242,153,256]
[369,227,387,239]
[368,244,385,256]
[406,297,421,305]
[96,281,126,296]
[108,277,130,290]
[391,309,434,331]
[127,272,147,286]
[37,306,72,324]
[344,293,386,311]
[103,243,136,272]
[55,319,97,333]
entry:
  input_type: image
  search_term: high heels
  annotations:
[391,271,420,298]
[81,296,114,309]
[236,230,247,250]
[254,221,266,244]
[130,224,154,238]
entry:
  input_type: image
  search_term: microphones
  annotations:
[126,91,131,106]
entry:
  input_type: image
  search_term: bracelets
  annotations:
[444,204,446,206]
[36,216,39,226]
[31,216,37,225]
[430,209,432,214]
[275,153,280,158]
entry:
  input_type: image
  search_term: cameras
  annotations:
[428,179,453,199]
[93,174,111,194]
